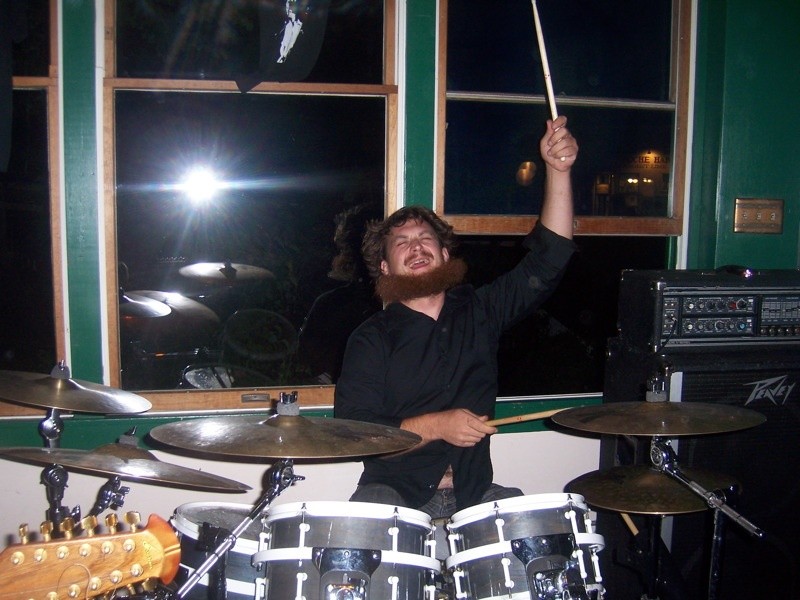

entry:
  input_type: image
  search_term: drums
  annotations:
[445,493,606,600]
[252,501,440,600]
[175,364,277,389]
[157,507,272,600]
[212,307,300,372]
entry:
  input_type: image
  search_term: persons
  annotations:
[331,117,579,520]
[284,205,381,386]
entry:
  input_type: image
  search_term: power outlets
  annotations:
[734,198,784,232]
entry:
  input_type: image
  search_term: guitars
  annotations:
[0,511,182,600]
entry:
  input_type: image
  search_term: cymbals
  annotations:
[564,465,743,515]
[127,288,217,322]
[149,414,422,461]
[120,291,172,318]
[550,403,768,437]
[180,262,276,282]
[0,445,252,491]
[0,368,153,413]
